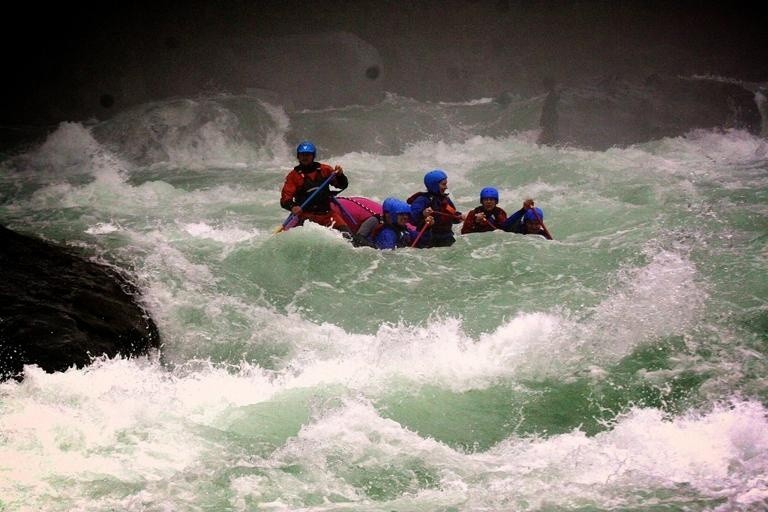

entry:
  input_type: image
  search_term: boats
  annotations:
[284,196,417,232]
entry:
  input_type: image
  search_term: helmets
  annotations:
[524,207,544,222]
[479,187,499,205]
[383,197,413,225]
[295,141,316,159]
[424,170,447,195]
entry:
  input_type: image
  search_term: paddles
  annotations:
[272,169,338,235]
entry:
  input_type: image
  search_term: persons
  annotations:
[353,196,399,249]
[504,198,549,239]
[280,143,356,244]
[461,186,506,233]
[369,197,436,253]
[409,170,464,250]
